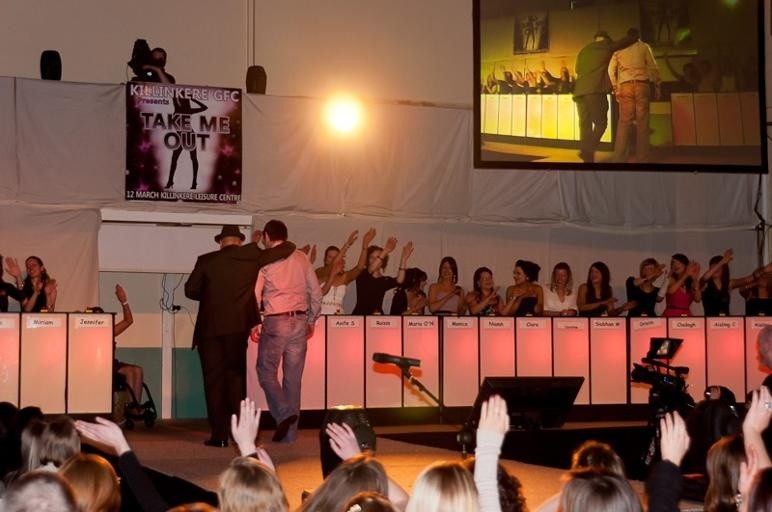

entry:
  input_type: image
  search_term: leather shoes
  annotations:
[272,415,297,442]
[205,439,228,447]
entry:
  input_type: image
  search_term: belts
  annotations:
[266,310,306,317]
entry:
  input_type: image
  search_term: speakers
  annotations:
[319,405,375,479]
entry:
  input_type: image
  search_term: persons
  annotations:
[0,384,772,512]
[250,218,323,443]
[0,254,24,313]
[519,12,539,53]
[164,87,208,191]
[573,31,641,162]
[132,47,176,83]
[480,58,572,94]
[667,61,702,95]
[608,28,662,164]
[185,223,297,448]
[85,283,145,420]
[21,256,57,313]
[300,227,772,317]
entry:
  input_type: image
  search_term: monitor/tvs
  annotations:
[472,0,768,172]
[464,377,585,428]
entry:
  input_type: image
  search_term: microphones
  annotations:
[373,352,420,367]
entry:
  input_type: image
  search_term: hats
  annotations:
[215,225,245,244]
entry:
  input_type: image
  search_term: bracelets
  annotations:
[121,301,128,306]
[16,280,24,288]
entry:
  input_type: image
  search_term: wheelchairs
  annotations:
[113,370,159,433]
